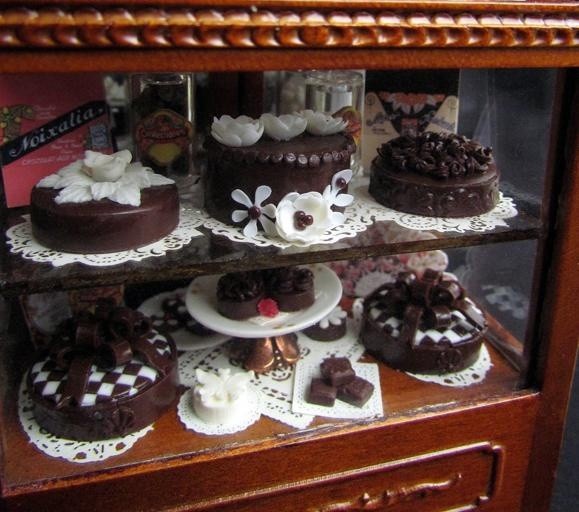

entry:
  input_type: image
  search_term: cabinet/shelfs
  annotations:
[0,0,579,512]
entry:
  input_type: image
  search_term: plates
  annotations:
[137,287,231,351]
[186,263,345,341]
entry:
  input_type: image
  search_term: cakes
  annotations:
[27,297,179,442]
[30,149,179,254]
[149,265,315,337]
[308,357,375,408]
[304,306,348,341]
[361,269,488,375]
[192,367,255,425]
[369,131,499,217]
[204,110,356,242]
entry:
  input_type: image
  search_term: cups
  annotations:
[304,70,367,177]
[126,72,194,191]
